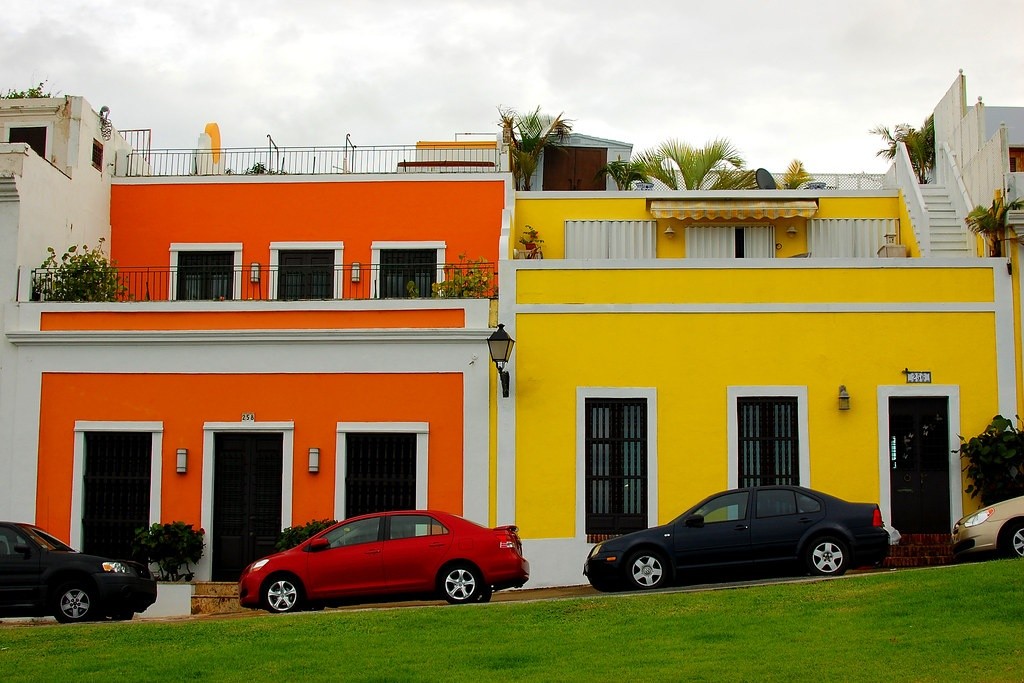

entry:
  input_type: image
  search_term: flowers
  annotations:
[519,225,545,244]
[133,521,206,582]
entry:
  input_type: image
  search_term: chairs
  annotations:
[756,168,777,190]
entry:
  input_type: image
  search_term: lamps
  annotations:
[785,224,798,238]
[308,447,319,472]
[251,261,259,282]
[838,385,850,410]
[176,447,187,472]
[350,261,361,281]
[487,323,516,398]
[664,225,674,234]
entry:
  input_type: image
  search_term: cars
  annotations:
[583,484,891,592]
[950,494,1024,559]
[237,509,528,614]
[0,521,157,624]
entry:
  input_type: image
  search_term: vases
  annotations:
[635,183,654,191]
[134,582,198,617]
[525,243,536,250]
